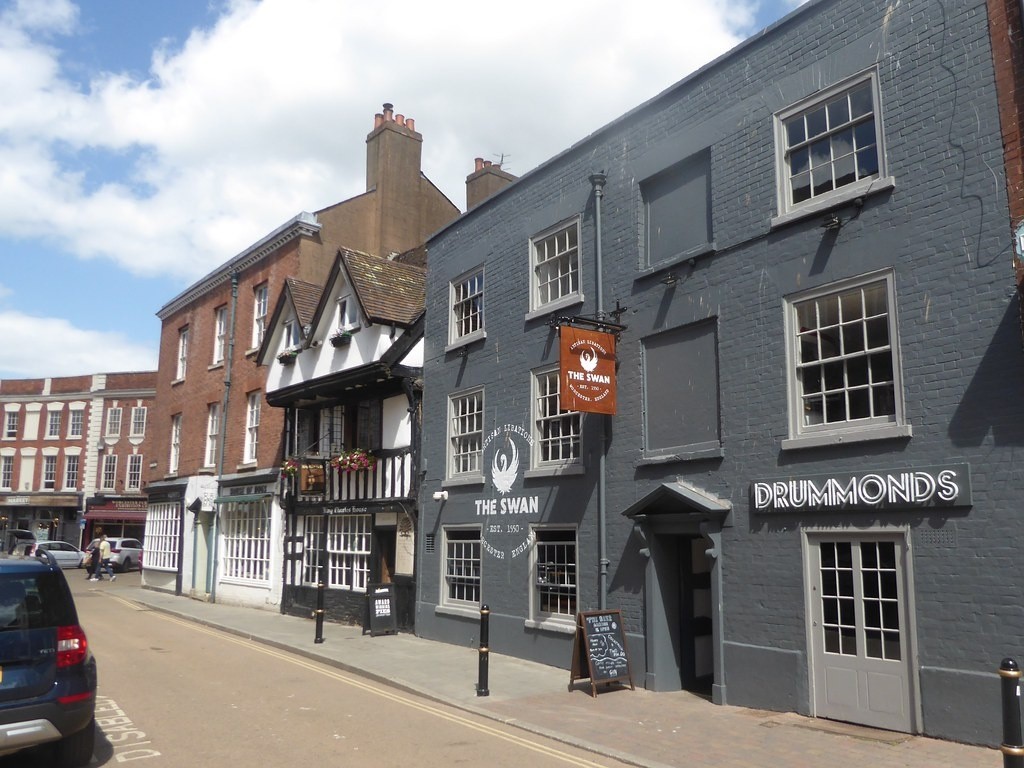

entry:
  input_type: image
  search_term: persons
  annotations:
[139,546,144,574]
[85,534,116,582]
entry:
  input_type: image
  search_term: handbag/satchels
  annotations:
[83,553,92,565]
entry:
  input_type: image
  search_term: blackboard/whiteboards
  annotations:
[570,608,632,682]
[365,582,399,633]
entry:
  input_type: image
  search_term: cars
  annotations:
[82,536,144,573]
[3,529,89,569]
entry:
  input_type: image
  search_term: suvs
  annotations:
[0,549,99,757]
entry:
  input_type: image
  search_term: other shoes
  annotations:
[90,578,100,582]
[85,577,90,579]
[110,576,116,582]
